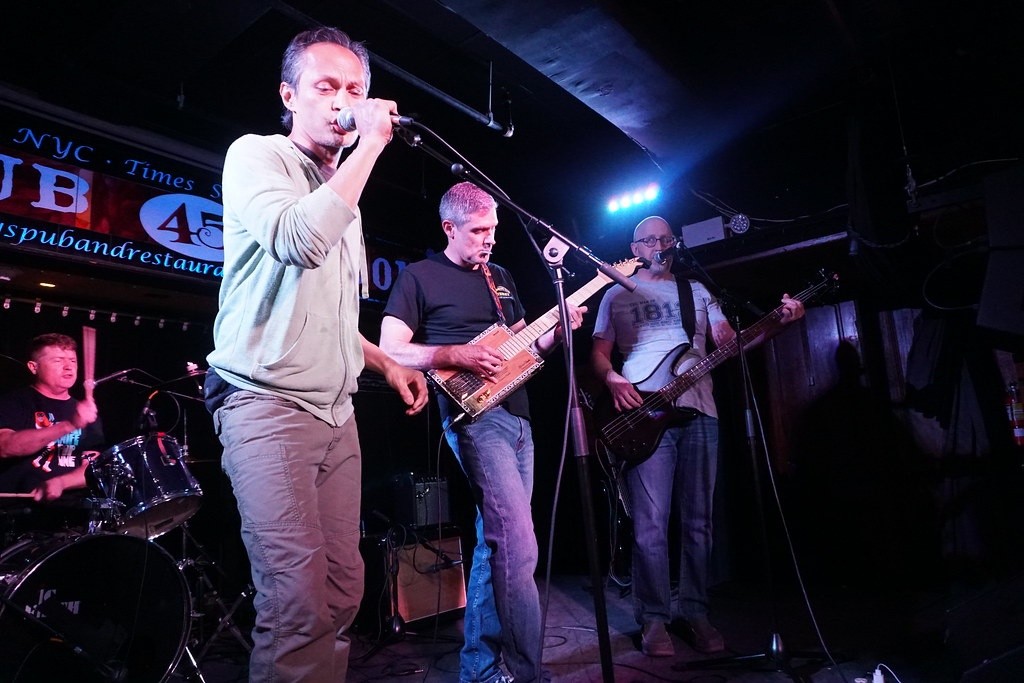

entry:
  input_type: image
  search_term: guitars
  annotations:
[425,255,652,425]
[591,265,846,470]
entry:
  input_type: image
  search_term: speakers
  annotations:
[973,164,1024,352]
[352,475,470,635]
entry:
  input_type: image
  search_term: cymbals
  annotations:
[184,457,221,464]
[139,368,209,397]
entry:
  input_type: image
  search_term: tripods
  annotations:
[676,248,858,683]
[176,407,254,658]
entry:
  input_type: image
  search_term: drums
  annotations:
[83,433,206,541]
[0,530,194,683]
[0,507,52,567]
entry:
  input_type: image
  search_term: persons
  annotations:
[205,27,430,683]
[591,215,806,656]
[378,182,590,683]
[0,333,108,521]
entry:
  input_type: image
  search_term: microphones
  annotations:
[83,368,133,390]
[654,242,683,264]
[337,106,414,132]
[429,560,464,573]
[139,399,152,429]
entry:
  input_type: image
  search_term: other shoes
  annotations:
[640,621,675,656]
[687,613,725,653]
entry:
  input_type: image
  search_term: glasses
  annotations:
[634,236,673,248]
